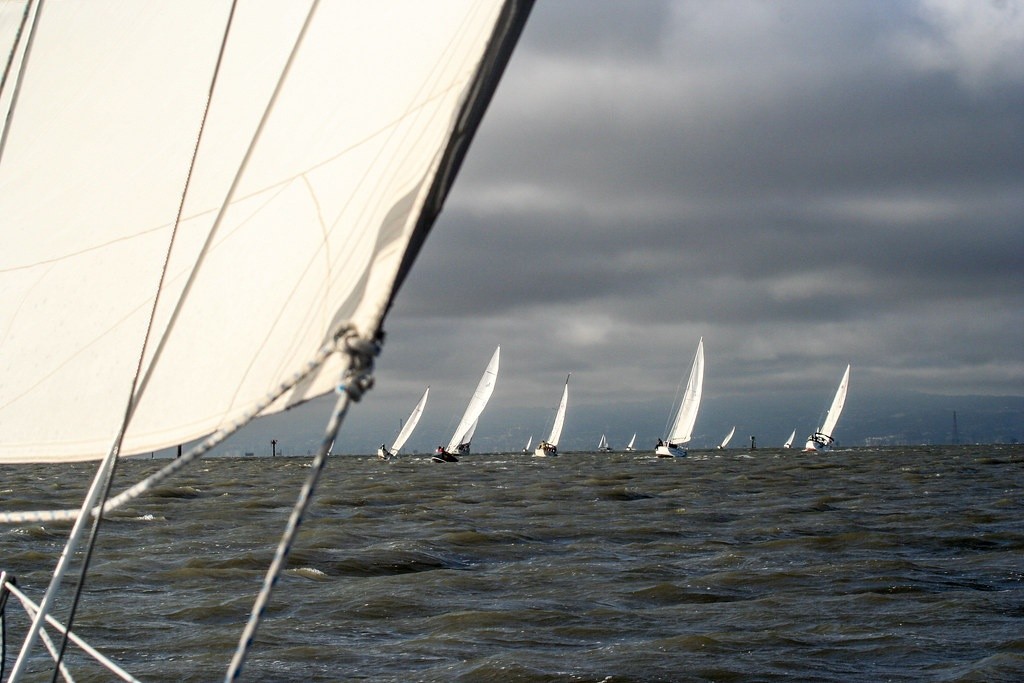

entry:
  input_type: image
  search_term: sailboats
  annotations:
[522,436,533,455]
[378,384,430,462]
[597,434,610,452]
[532,373,570,458]
[784,429,797,448]
[626,432,638,451]
[806,364,850,451]
[655,336,705,459]
[716,426,736,451]
[431,345,501,463]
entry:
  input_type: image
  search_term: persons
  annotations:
[436,446,444,452]
[541,441,546,448]
[655,438,663,451]
